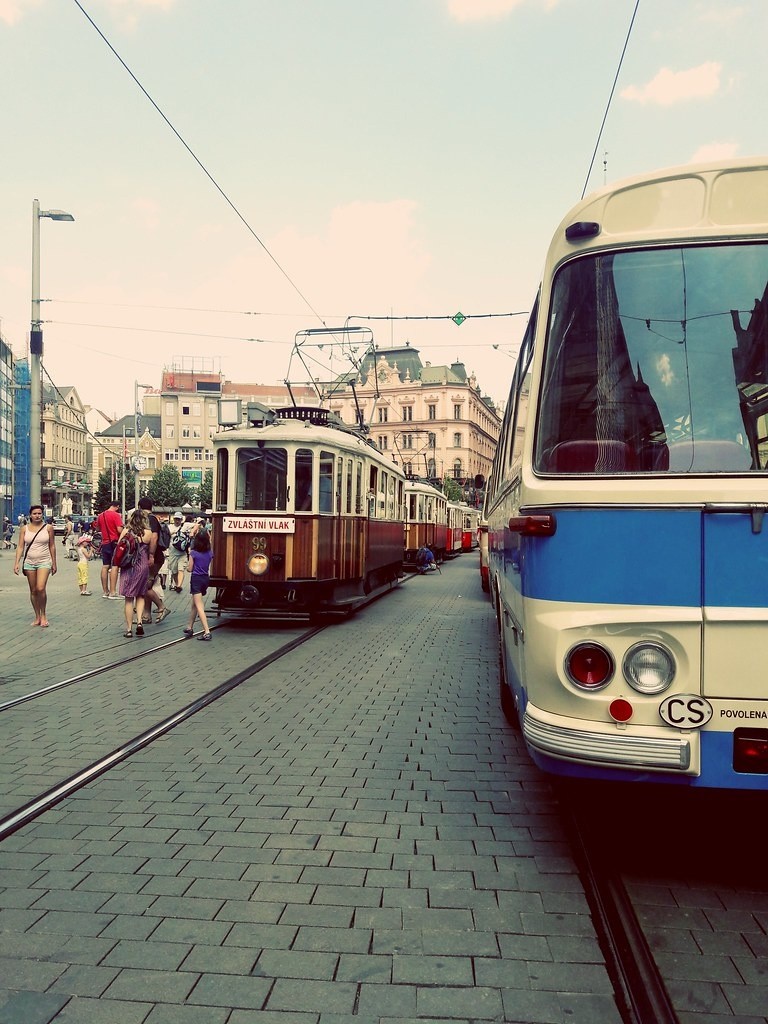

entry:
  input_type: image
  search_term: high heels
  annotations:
[163,585,166,590]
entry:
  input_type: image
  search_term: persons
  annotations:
[183,530,211,641]
[96,500,126,601]
[299,465,333,512]
[132,496,171,623]
[153,513,213,591]
[75,536,94,596]
[416,542,437,575]
[0,512,96,559]
[115,509,153,638]
[12,503,58,629]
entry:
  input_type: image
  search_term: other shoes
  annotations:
[14,544,17,550]
[174,585,182,593]
[419,571,428,575]
[40,618,49,627]
[8,544,11,549]
[31,617,40,626]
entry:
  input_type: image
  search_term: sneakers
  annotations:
[182,628,193,635]
[80,590,92,595]
[197,633,212,640]
[102,591,109,598]
[107,591,125,599]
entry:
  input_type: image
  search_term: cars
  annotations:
[53,519,74,533]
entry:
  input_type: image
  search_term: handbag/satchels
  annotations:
[172,535,187,552]
[21,560,26,575]
[111,532,137,569]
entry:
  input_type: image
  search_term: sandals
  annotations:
[135,623,144,635]
[154,607,171,624]
[132,616,152,623]
[123,630,133,637]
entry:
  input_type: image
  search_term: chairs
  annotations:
[536,437,754,471]
[416,547,443,576]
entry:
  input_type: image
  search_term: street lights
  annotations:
[122,427,135,526]
[29,208,77,503]
[135,384,154,509]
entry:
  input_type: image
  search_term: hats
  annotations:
[77,536,91,546]
[173,512,182,519]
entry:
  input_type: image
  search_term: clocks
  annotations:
[134,456,146,471]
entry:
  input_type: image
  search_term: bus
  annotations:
[366,490,399,520]
[446,505,480,553]
[204,400,404,624]
[404,481,449,565]
[477,156,767,820]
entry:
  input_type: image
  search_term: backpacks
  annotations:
[9,526,15,533]
[148,514,171,551]
[415,549,426,566]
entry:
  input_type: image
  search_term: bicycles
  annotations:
[91,533,102,557]
